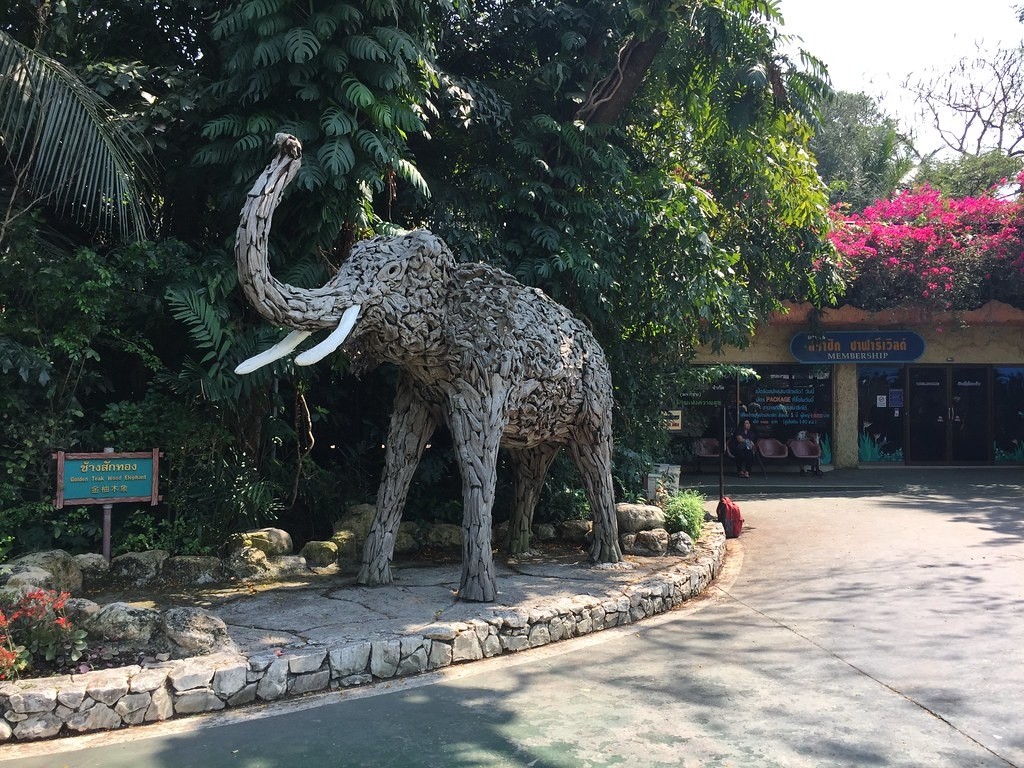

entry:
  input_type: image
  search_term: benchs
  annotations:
[694,437,821,477]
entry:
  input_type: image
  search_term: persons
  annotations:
[729,418,755,478]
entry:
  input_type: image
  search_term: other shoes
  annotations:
[739,471,750,478]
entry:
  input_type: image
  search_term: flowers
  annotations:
[800,169,1024,332]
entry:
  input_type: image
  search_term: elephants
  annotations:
[231,130,623,602]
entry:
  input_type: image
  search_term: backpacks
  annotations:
[718,498,744,538]
